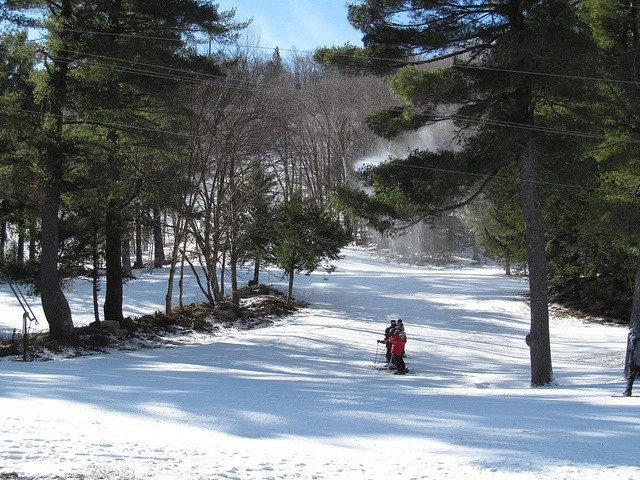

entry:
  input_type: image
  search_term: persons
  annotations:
[378,316,407,375]
